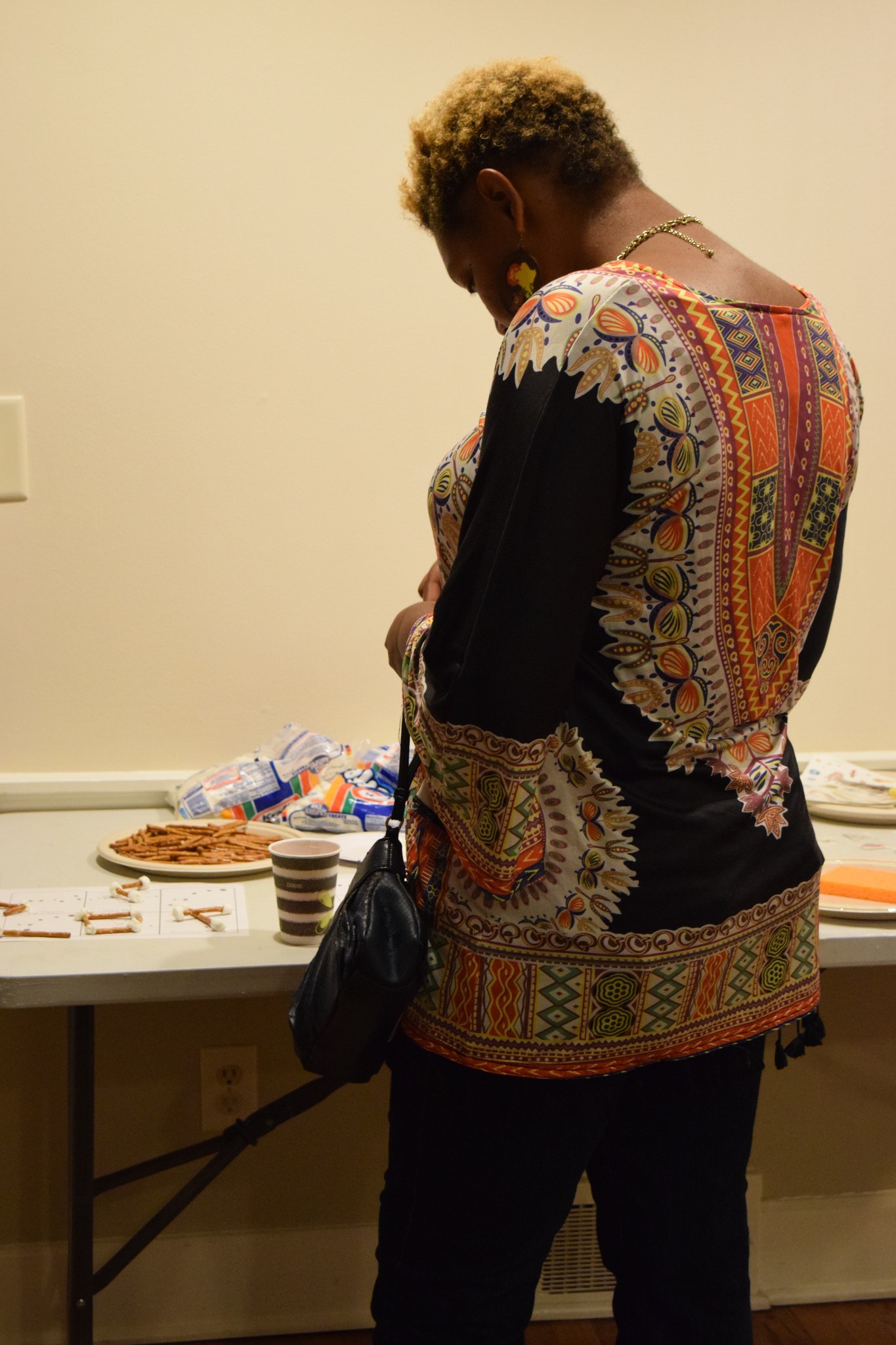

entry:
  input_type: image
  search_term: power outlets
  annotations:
[197,1042,257,1142]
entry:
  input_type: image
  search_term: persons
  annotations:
[365,59,866,1345]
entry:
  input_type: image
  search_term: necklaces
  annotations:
[616,214,715,261]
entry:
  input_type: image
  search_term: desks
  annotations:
[0,805,896,1345]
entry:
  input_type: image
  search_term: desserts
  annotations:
[815,866,896,903]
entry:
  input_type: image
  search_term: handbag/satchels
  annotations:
[287,623,430,1085]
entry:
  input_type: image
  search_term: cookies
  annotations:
[110,820,286,864]
[0,875,233,937]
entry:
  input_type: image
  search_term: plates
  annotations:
[817,860,896,920]
[329,831,407,863]
[97,819,307,877]
[805,801,896,824]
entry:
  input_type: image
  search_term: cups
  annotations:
[267,838,341,944]
